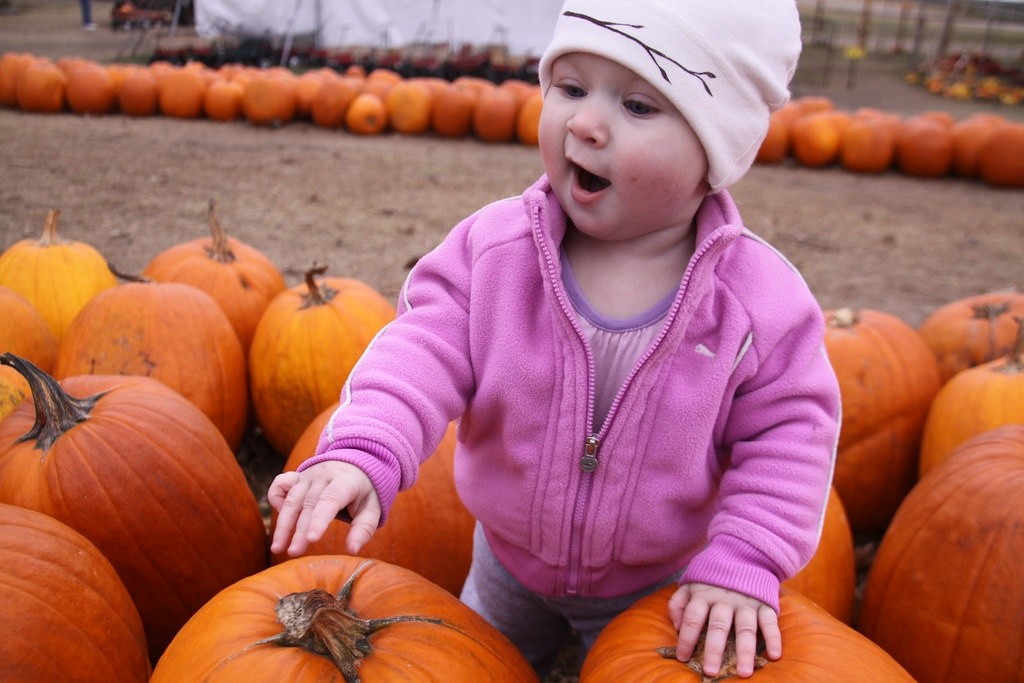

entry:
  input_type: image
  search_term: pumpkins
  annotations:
[0,54,1024,683]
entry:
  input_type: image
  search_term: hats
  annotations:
[538,0,802,198]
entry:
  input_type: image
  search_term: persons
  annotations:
[266,0,841,683]
